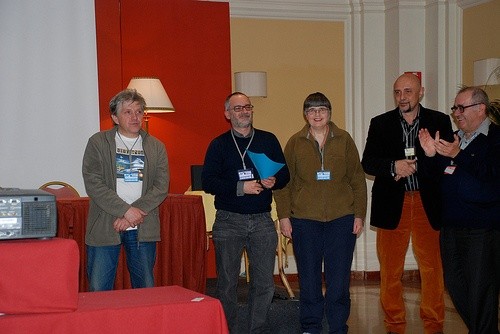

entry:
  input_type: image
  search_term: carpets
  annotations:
[206,276,330,334]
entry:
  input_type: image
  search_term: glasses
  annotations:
[451,103,485,113]
[228,104,253,112]
[306,108,330,114]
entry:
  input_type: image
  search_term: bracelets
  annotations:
[391,160,396,175]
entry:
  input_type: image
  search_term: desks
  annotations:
[184,185,295,298]
[0,285,229,334]
[56,194,207,295]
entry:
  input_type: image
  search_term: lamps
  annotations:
[234,71,267,99]
[126,77,175,133]
[472,57,500,86]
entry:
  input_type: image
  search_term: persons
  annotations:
[361,73,455,334]
[418,86,500,334]
[82,88,169,292]
[273,92,368,334]
[201,92,290,334]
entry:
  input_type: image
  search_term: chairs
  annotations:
[39,181,80,197]
